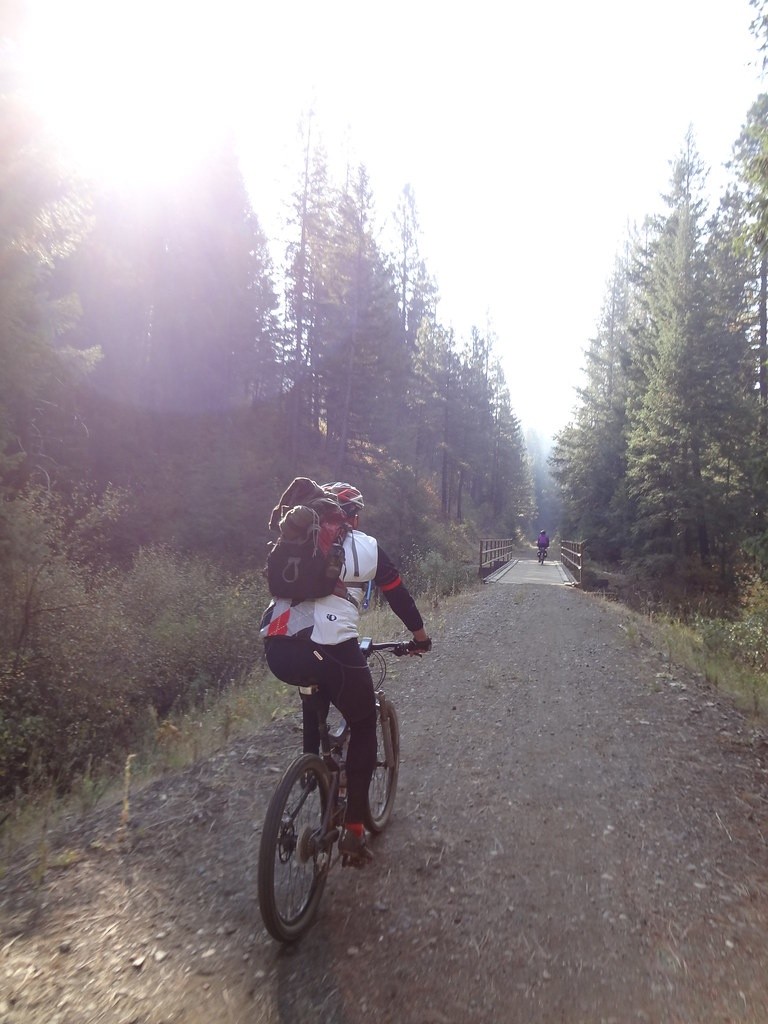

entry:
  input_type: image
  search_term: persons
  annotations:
[537,529,549,562]
[258,479,438,866]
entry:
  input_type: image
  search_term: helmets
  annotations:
[540,530,547,535]
[317,481,366,517]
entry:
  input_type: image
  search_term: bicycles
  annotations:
[537,546,547,565]
[256,636,432,944]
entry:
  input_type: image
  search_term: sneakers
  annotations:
[337,822,374,862]
[302,769,317,791]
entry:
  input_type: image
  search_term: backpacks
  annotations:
[267,477,359,606]
